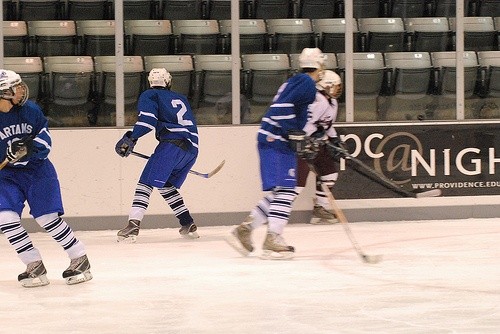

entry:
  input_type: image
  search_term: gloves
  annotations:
[288,130,306,141]
[312,127,324,139]
[5,136,31,163]
[328,137,341,152]
[115,131,137,157]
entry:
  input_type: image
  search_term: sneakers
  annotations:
[179,222,200,239]
[259,232,296,260]
[17,260,49,287]
[115,220,140,245]
[310,206,339,224]
[225,215,255,256]
[62,253,93,285]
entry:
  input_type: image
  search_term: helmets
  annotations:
[148,67,173,88]
[299,47,327,70]
[315,70,342,98]
[0,69,29,107]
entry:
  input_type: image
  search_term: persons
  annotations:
[292,70,341,225]
[0,69,93,286]
[115,68,200,242]
[225,48,327,259]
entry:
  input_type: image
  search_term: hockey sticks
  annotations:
[326,145,442,199]
[129,149,225,178]
[307,160,385,265]
[0,132,32,171]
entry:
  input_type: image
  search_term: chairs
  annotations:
[0,0,500,127]
